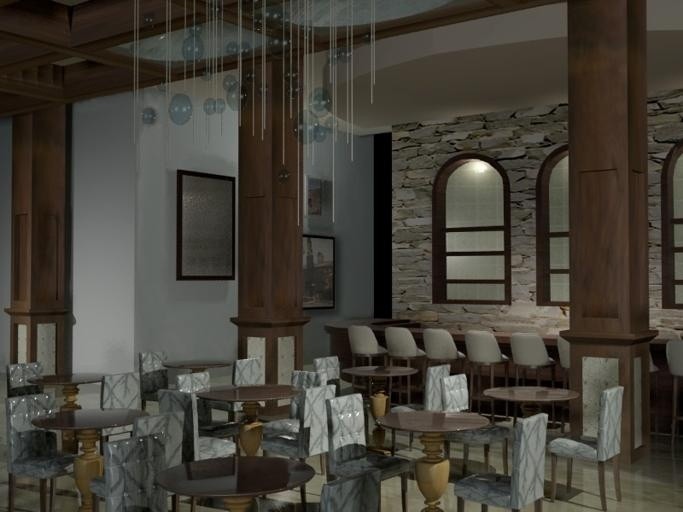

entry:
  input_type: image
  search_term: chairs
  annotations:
[96,372,141,461]
[5,364,43,395]
[103,431,168,511]
[454,412,544,512]
[387,363,451,456]
[438,374,488,461]
[4,394,81,509]
[318,471,380,512]
[134,413,185,510]
[157,390,238,512]
[313,356,366,436]
[139,352,169,412]
[547,385,624,510]
[325,395,410,511]
[179,373,241,452]
[209,358,261,422]
[264,372,324,475]
[258,386,335,504]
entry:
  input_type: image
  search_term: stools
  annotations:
[557,330,571,426]
[466,332,508,427]
[510,332,555,426]
[383,327,424,406]
[649,351,659,440]
[424,329,467,376]
[348,325,385,395]
[666,334,683,443]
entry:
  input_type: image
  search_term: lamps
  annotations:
[129,1,378,169]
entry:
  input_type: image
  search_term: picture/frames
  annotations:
[174,169,236,280]
[301,232,336,313]
[302,173,335,225]
